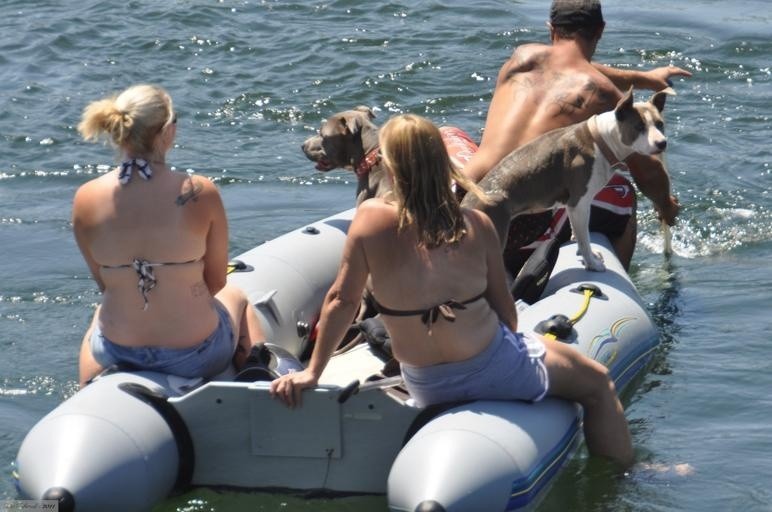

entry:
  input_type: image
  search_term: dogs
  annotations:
[457,86,679,295]
[298,103,507,323]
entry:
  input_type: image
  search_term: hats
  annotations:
[548,0,601,26]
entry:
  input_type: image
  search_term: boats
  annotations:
[11,205,662,512]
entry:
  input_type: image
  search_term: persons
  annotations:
[455,0,695,274]
[70,79,273,402]
[268,114,695,487]
